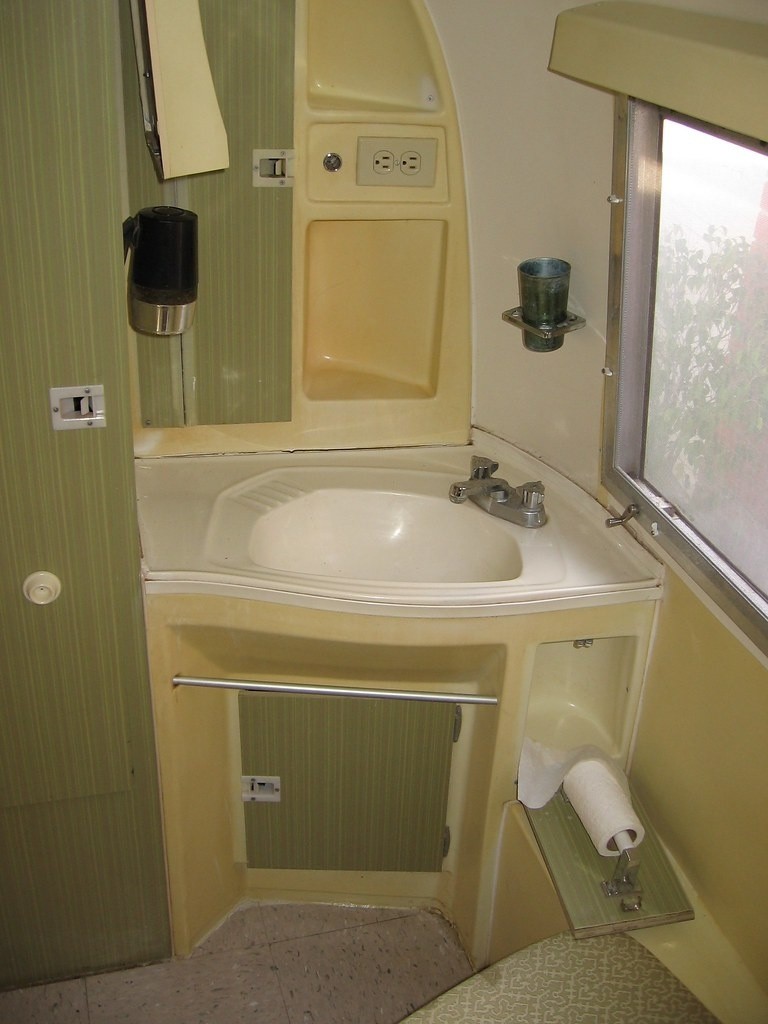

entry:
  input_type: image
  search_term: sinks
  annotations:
[202,465,567,591]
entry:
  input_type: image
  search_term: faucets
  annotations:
[448,476,512,504]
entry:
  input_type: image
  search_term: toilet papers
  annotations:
[516,736,646,857]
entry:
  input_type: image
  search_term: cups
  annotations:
[517,259,572,352]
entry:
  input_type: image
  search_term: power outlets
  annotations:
[356,137,437,188]
